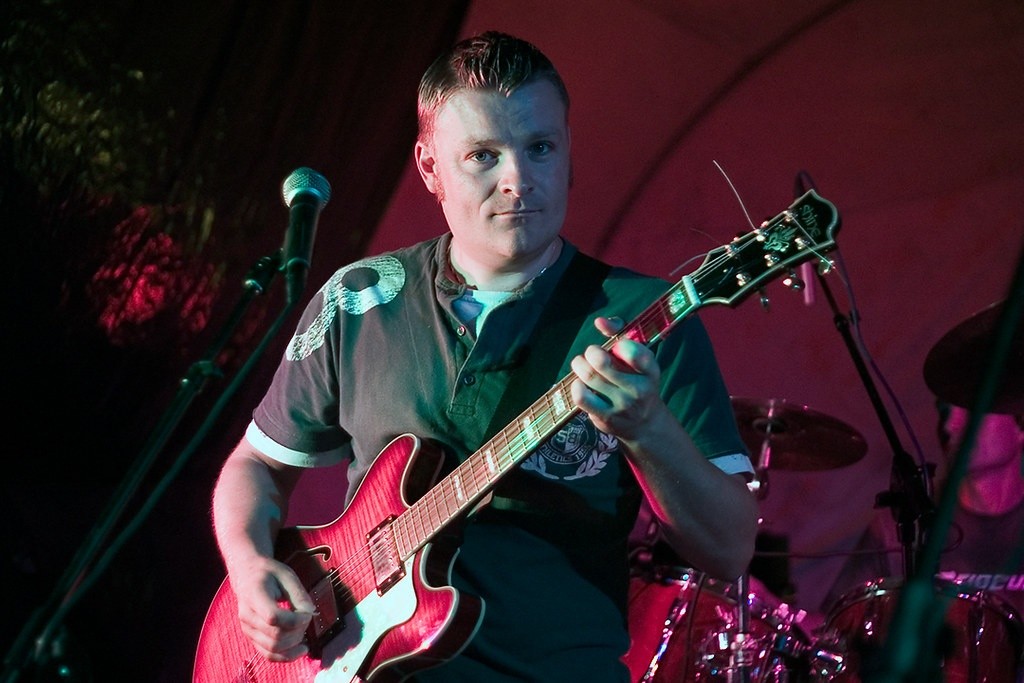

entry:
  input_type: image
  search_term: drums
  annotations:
[619,569,811,683]
[823,575,1024,683]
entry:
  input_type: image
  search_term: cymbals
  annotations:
[922,299,1024,416]
[727,394,868,471]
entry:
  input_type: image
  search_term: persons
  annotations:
[210,32,759,683]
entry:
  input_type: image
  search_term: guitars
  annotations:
[192,187,842,683]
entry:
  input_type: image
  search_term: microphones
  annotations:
[281,166,333,306]
[794,175,816,307]
